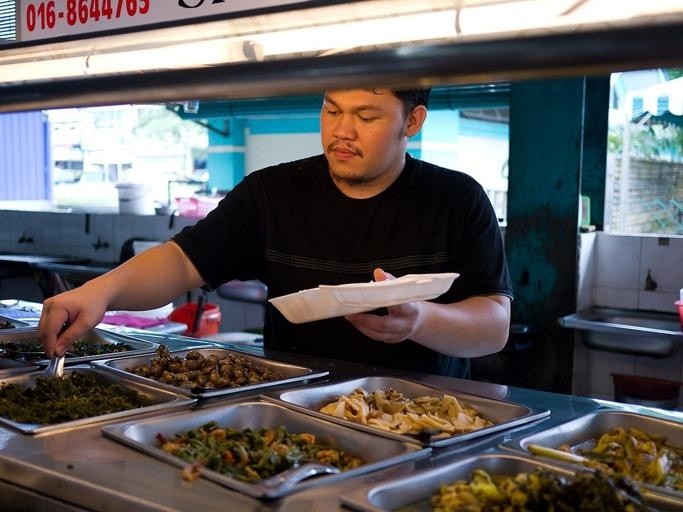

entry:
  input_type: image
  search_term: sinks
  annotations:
[560,304,683,358]
[47,258,112,284]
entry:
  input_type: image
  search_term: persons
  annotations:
[38,86,514,378]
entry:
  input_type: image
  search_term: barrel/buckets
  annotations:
[172,291,223,340]
[608,371,682,411]
[115,182,146,216]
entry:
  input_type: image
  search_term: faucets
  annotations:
[643,267,660,293]
[90,235,110,251]
[16,228,35,245]
